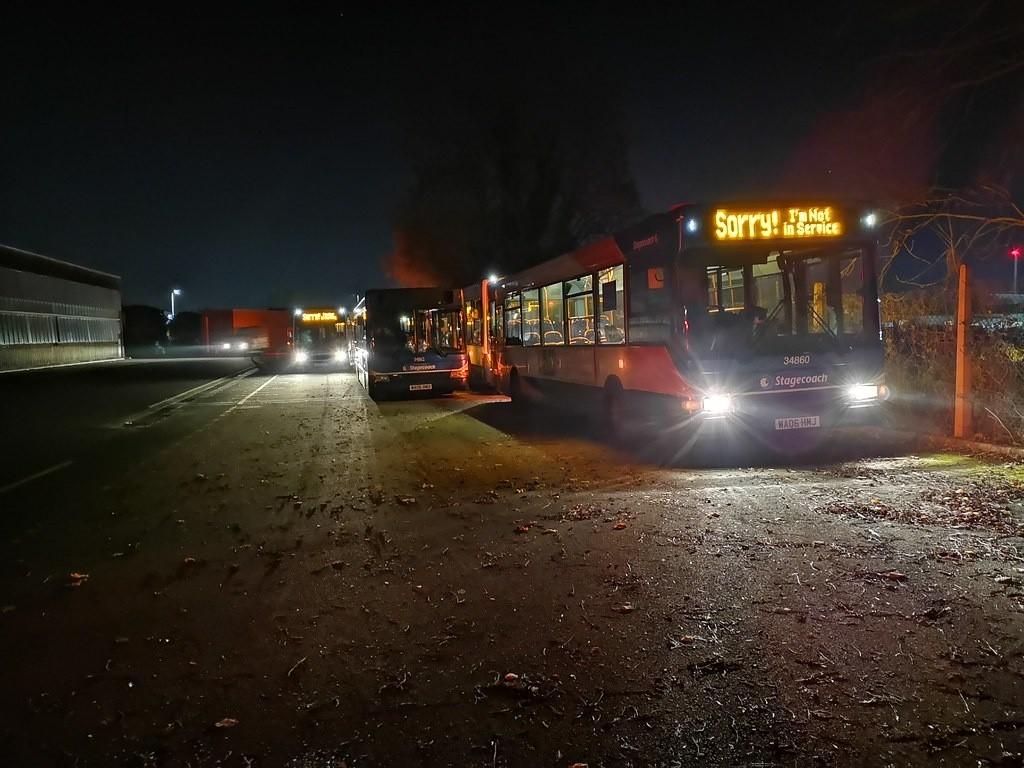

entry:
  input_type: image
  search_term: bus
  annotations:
[292,307,350,372]
[464,200,886,453]
[346,286,470,401]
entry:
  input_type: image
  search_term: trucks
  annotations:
[219,326,270,355]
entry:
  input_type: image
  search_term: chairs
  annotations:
[506,314,623,344]
